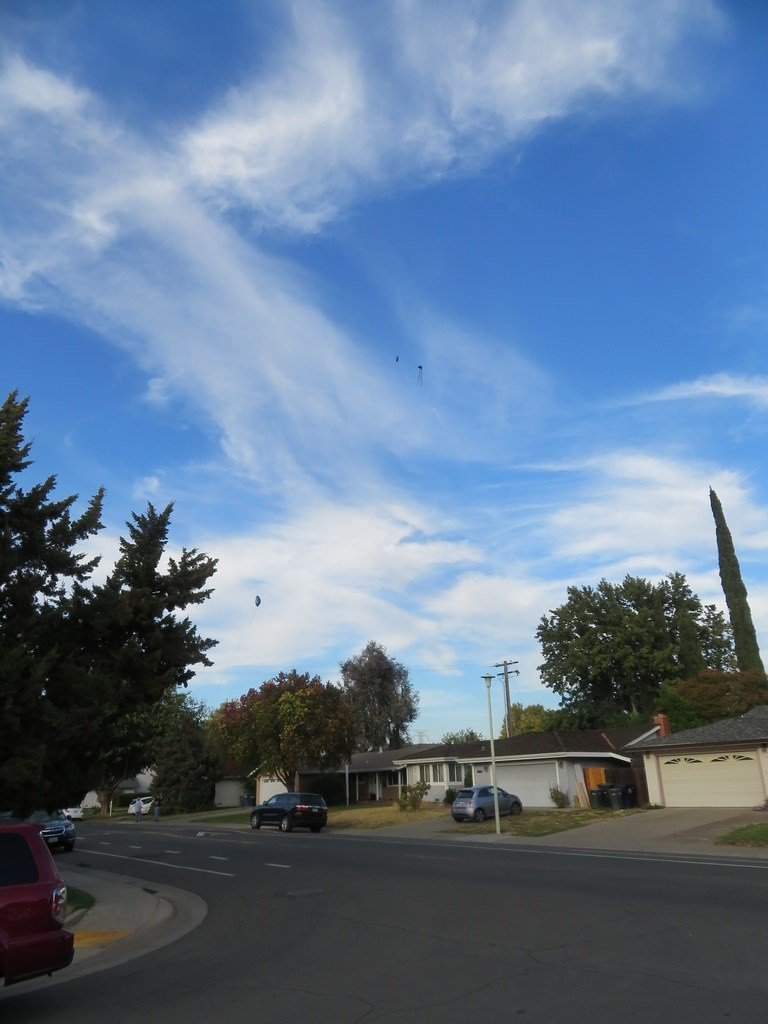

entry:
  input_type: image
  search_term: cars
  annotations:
[452,786,523,823]
[128,797,155,816]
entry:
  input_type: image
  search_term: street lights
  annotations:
[481,672,501,835]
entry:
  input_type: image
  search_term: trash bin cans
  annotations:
[240,795,248,807]
[248,794,256,806]
[598,783,636,808]
[589,789,602,809]
[609,788,624,810]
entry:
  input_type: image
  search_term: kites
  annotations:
[254,595,261,607]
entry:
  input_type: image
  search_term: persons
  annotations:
[153,793,163,822]
[132,797,143,822]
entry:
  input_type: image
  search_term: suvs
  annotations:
[0,805,84,852]
[0,825,74,988]
[250,793,328,833]
